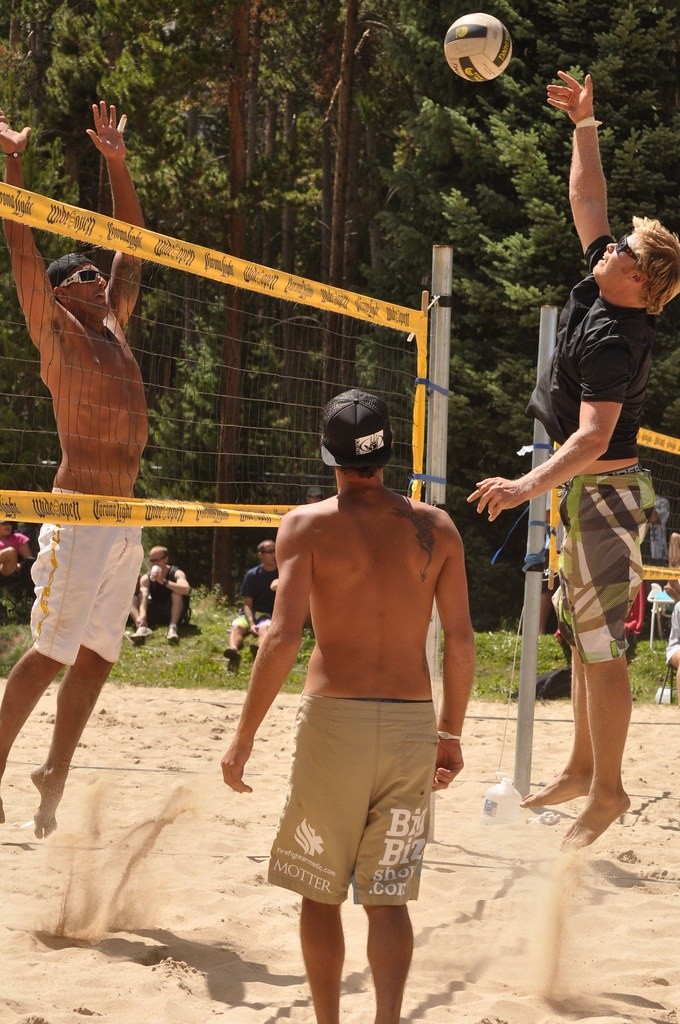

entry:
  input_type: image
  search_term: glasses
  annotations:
[614,233,642,271]
[1,521,12,525]
[59,268,102,287]
[148,556,166,563]
[263,549,276,555]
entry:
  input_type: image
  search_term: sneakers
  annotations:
[129,627,153,642]
[166,626,178,642]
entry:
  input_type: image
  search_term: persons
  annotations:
[0,520,32,586]
[223,540,279,660]
[0,101,149,840]
[466,70,680,852]
[509,482,680,704]
[221,387,476,1024]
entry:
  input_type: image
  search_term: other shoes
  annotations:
[224,648,241,661]
[250,645,260,660]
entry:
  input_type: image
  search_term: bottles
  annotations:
[480,778,522,843]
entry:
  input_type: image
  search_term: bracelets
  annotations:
[163,579,168,586]
[126,547,189,640]
[576,114,602,127]
[437,731,461,740]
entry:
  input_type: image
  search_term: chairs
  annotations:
[647,533,680,704]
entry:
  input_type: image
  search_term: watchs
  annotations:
[4,152,23,158]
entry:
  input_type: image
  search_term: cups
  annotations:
[150,565,162,581]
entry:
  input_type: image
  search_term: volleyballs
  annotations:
[444,10,516,81]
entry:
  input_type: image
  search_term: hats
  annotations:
[45,253,95,289]
[319,389,393,467]
